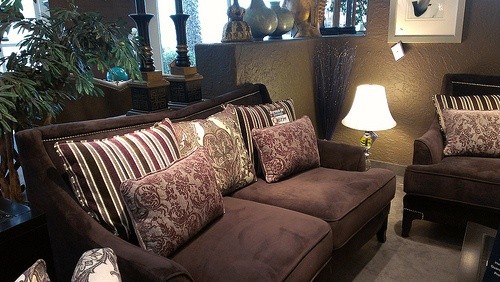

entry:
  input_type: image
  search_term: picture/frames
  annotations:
[388,0,467,43]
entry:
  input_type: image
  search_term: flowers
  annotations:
[312,39,358,140]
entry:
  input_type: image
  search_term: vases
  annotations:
[243,0,279,41]
[269,2,294,39]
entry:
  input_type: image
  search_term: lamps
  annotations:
[342,84,397,170]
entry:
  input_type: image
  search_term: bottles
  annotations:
[269,1,295,39]
[243,0,278,40]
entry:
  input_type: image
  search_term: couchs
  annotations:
[402,73,500,239]
[16,84,396,282]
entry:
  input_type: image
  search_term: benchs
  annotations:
[63,75,133,123]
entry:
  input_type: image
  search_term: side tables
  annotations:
[0,199,54,282]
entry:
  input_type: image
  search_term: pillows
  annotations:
[173,103,257,195]
[235,97,296,178]
[55,117,182,241]
[432,93,500,137]
[441,108,500,158]
[252,114,321,183]
[121,147,225,258]
